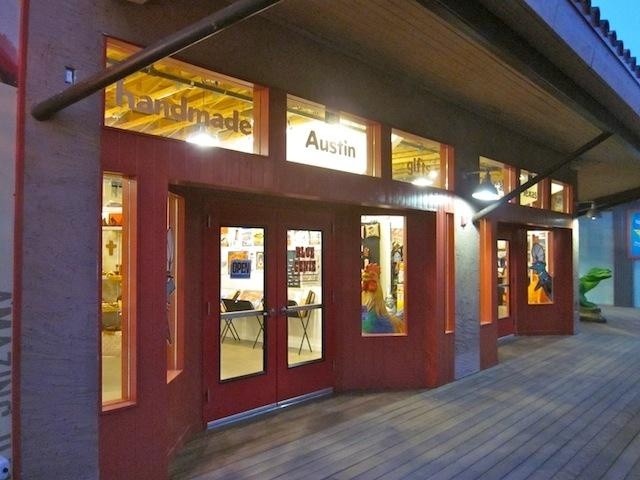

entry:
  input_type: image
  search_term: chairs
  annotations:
[221,288,316,356]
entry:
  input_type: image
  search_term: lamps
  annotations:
[575,200,603,220]
[462,167,500,201]
